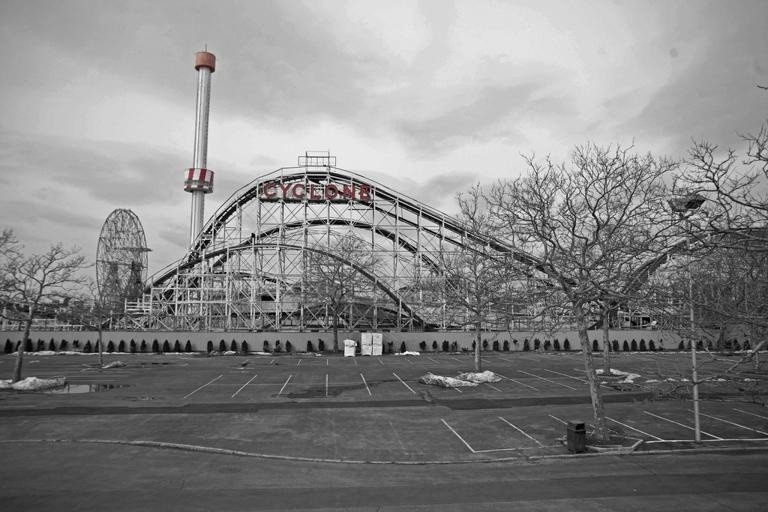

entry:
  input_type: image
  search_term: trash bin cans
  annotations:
[567,422,586,454]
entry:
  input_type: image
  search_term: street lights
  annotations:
[668,193,705,444]
